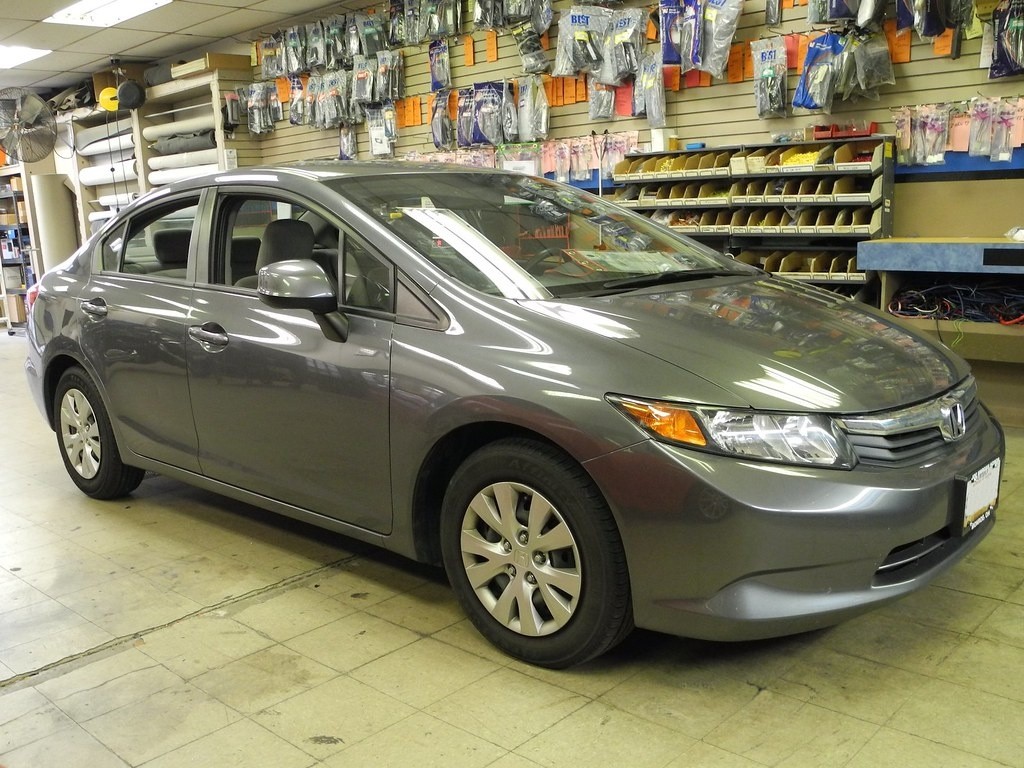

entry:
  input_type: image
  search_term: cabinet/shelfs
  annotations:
[613,133,898,286]
[68,71,253,249]
[0,129,45,334]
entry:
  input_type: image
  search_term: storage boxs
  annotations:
[92,64,151,102]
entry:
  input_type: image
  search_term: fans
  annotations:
[0,87,57,164]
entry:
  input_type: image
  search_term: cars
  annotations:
[22,148,1010,671]
